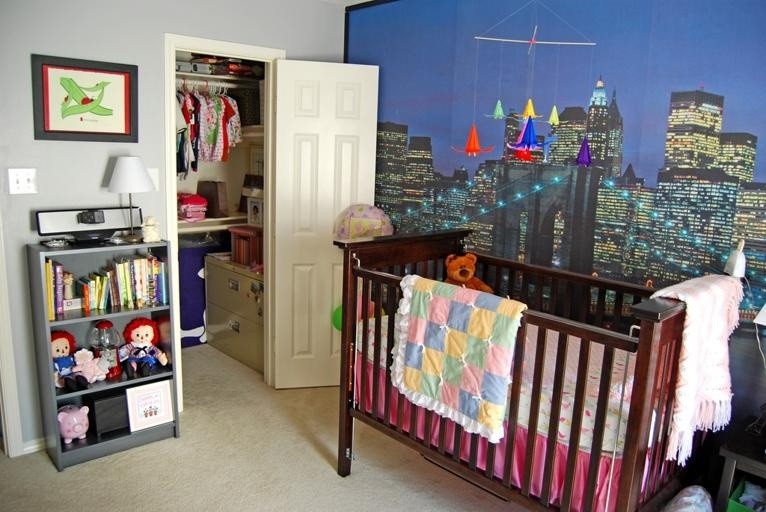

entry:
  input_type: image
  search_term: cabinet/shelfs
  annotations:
[25,240,179,472]
[205,256,264,375]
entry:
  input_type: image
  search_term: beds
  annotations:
[333,229,744,512]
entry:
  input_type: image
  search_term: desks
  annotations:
[717,416,766,512]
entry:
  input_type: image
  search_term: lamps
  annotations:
[108,156,156,242]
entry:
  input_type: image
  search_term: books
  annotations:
[230,231,262,266]
[42,254,169,320]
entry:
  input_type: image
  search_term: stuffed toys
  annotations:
[442,252,493,294]
[70,341,110,381]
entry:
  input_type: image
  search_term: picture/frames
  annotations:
[247,198,263,229]
[30,53,138,143]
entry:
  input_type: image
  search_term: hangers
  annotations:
[176,77,227,100]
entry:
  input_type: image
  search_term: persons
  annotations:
[115,317,170,379]
[155,318,171,370]
[42,328,88,392]
[250,203,260,225]
